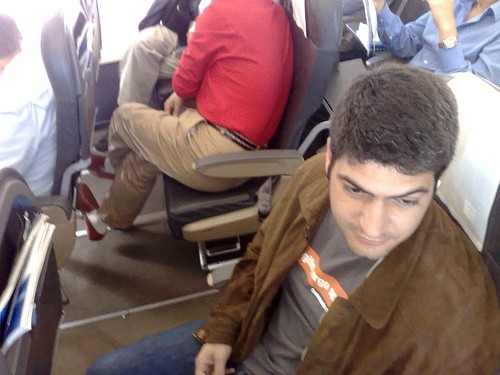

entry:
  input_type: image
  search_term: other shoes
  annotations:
[94,137,108,151]
[255,191,271,215]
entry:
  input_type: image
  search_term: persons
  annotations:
[76,0,294,240]
[372,0,500,88]
[0,14,57,196]
[85,64,500,375]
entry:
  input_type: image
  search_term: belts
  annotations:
[209,122,267,150]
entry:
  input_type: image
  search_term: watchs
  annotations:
[438,36,459,49]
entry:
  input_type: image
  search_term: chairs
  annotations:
[40,6,91,269]
[164,0,344,256]
[345,1,431,67]
[182,71,500,287]
[1,170,63,374]
[73,1,102,84]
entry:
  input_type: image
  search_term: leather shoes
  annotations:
[76,183,105,240]
[87,155,115,179]
